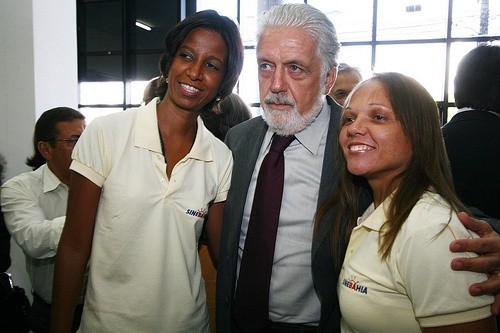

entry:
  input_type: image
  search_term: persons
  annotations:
[216,3,500,333]
[0,107,91,333]
[440,46,500,221]
[337,72,495,333]
[141,76,252,333]
[328,63,362,107]
[51,10,244,333]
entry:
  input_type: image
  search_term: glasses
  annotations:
[46,135,78,144]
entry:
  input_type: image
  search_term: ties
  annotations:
[229,134,296,333]
